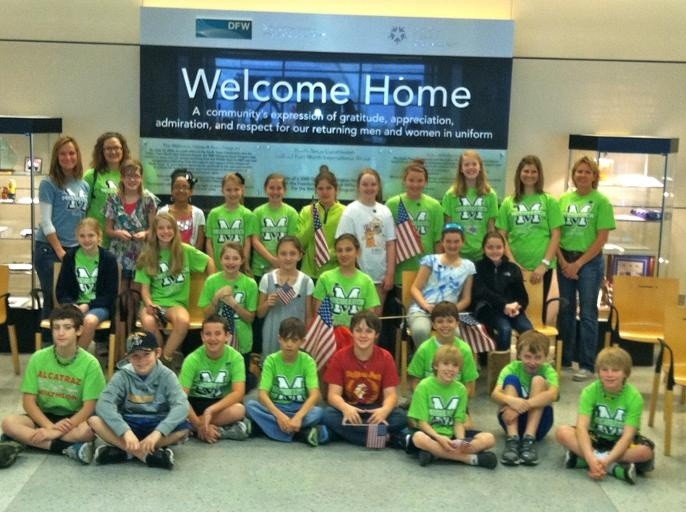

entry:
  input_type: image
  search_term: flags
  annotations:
[367,424,386,449]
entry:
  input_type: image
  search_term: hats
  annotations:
[121,331,158,361]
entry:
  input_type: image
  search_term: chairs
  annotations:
[0,263,206,381]
[604,275,681,347]
[649,302,686,457]
[395,269,478,398]
[475,269,571,401]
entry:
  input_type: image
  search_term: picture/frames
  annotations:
[23,155,43,174]
[612,254,650,276]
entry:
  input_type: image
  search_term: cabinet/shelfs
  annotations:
[565,134,679,367]
[0,115,63,355]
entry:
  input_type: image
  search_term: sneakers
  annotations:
[62,442,93,465]
[613,462,636,484]
[573,369,594,382]
[549,360,573,370]
[0,441,22,469]
[96,343,107,354]
[389,432,432,466]
[292,426,332,447]
[500,436,521,465]
[93,445,127,465]
[159,352,183,373]
[520,435,539,464]
[145,447,175,469]
[564,451,588,468]
[477,452,496,469]
[216,421,249,440]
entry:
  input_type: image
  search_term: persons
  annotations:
[395,344,497,469]
[31,132,566,398]
[172,313,251,442]
[246,317,331,447]
[557,156,616,382]
[1,303,106,463]
[555,344,655,485]
[86,329,193,470]
[321,310,409,450]
[490,329,560,466]
